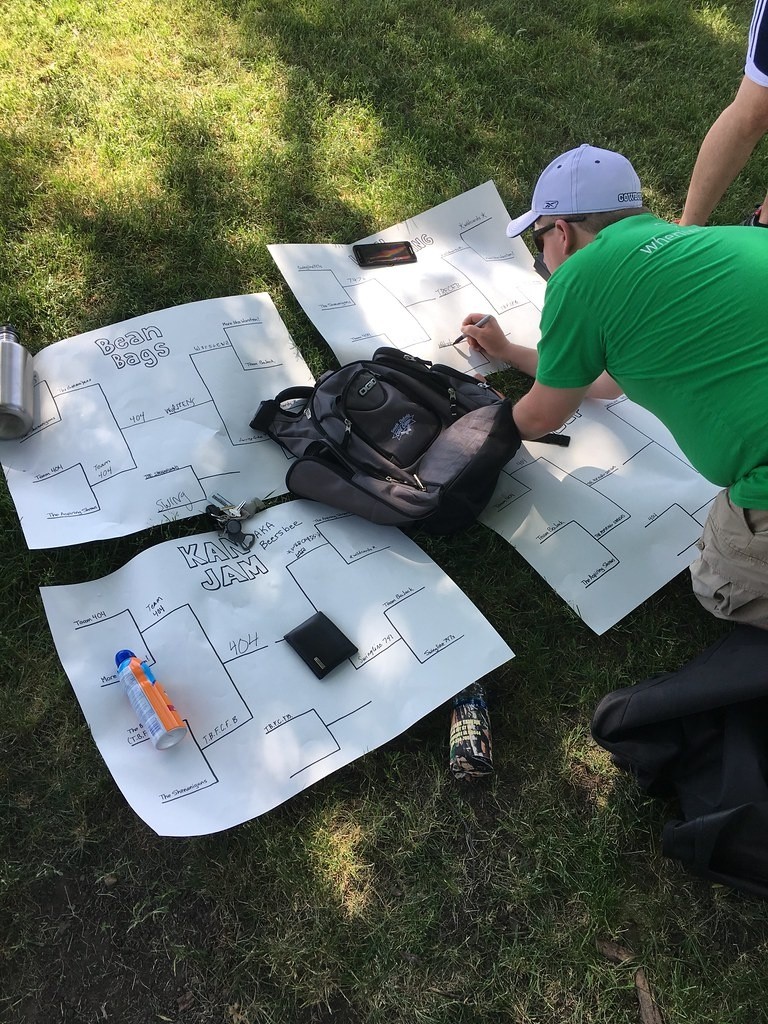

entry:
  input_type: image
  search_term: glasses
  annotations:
[532,215,587,255]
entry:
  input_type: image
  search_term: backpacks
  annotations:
[249,345,574,542]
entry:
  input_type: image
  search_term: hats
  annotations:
[506,144,642,239]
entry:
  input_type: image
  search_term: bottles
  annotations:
[115,650,187,750]
[0,325,34,440]
[451,683,493,788]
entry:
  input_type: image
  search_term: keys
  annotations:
[210,500,246,534]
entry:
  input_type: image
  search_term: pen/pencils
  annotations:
[451,314,492,345]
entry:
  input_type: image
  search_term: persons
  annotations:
[460,145,768,630]
[677,1,768,226]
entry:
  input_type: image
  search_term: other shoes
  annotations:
[736,204,762,226]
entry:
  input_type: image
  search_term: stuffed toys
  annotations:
[241,497,265,518]
